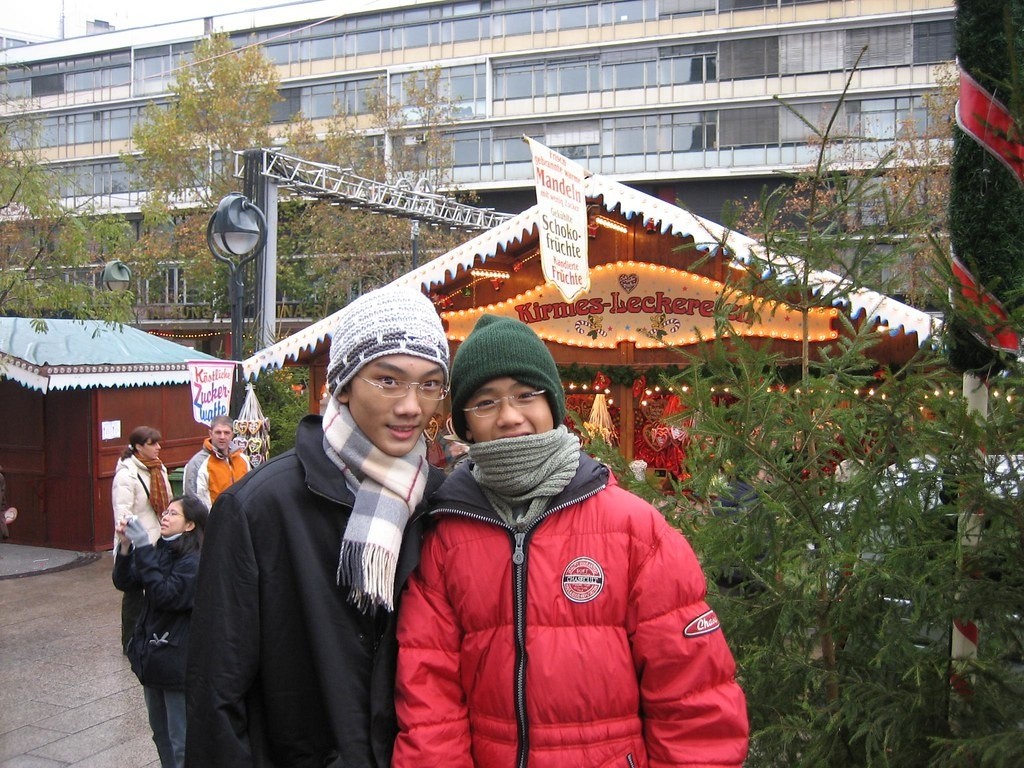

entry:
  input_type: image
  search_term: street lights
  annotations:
[206,190,269,360]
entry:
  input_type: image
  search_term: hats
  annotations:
[450,313,567,444]
[326,285,451,398]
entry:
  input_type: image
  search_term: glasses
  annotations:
[461,383,547,418]
[355,372,449,401]
[161,510,185,517]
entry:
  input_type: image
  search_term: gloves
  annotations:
[123,518,151,547]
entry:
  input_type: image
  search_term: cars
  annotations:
[808,454,1024,595]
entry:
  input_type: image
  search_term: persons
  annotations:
[112,426,173,565]
[182,282,452,768]
[112,493,210,768]
[706,459,773,599]
[183,414,249,514]
[391,313,749,768]
